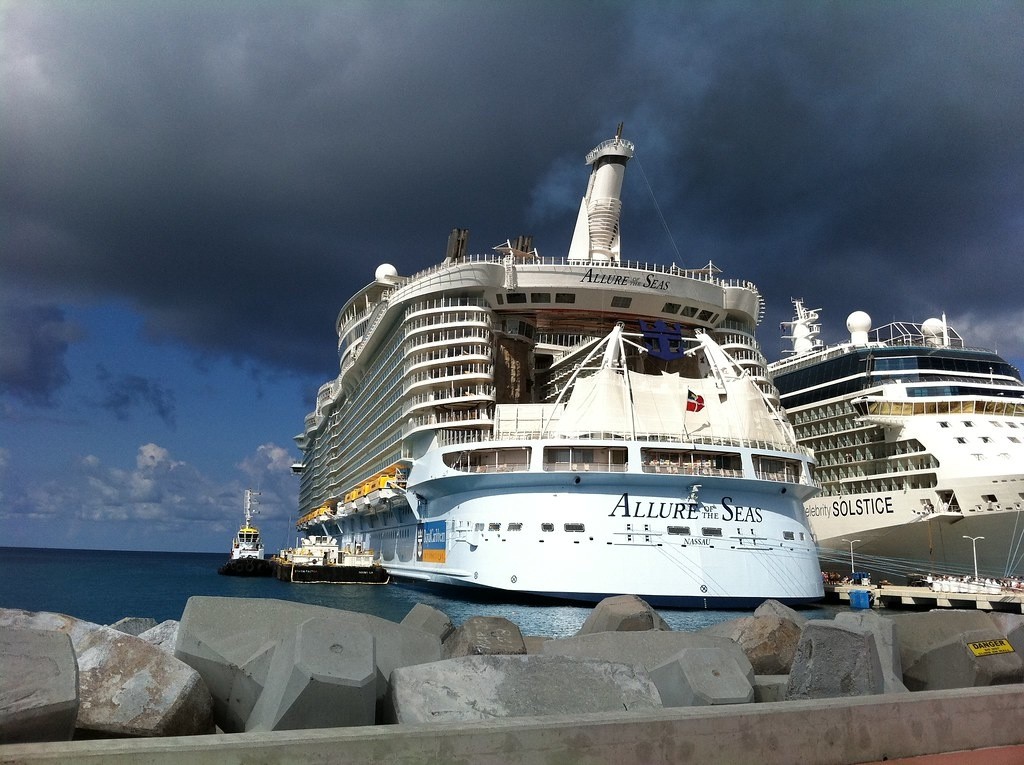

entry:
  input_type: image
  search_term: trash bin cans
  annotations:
[848,589,872,609]
[861,578,868,586]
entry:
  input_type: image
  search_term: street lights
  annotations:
[841,537,861,572]
[962,534,985,581]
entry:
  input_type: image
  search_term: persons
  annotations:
[868,572,872,587]
[821,571,853,586]
[866,591,877,610]
[927,573,932,590]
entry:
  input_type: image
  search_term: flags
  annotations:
[686,389,704,412]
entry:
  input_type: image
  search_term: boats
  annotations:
[271,528,390,585]
[215,488,274,577]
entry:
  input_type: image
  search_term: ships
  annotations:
[288,120,830,607]
[765,294,1024,600]
